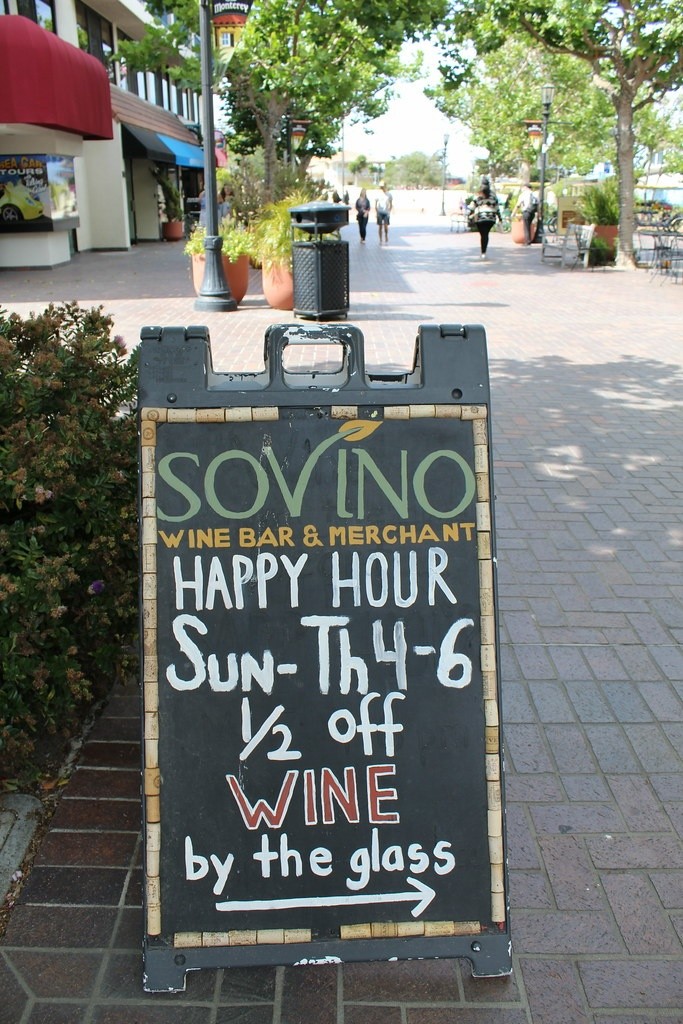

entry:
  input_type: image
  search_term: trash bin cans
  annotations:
[289,202,352,320]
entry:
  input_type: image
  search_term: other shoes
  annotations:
[480,253,486,259]
[360,238,365,244]
[525,239,532,246]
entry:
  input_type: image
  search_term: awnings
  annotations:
[122,123,205,168]
[0,15,114,140]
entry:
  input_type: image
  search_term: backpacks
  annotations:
[527,192,538,213]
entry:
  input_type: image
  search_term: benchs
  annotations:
[451,212,470,232]
[537,223,598,270]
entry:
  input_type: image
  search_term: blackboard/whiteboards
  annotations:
[131,321,507,952]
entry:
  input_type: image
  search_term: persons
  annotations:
[198,184,234,228]
[332,189,349,205]
[354,188,371,243]
[467,179,503,259]
[374,180,393,245]
[510,183,542,246]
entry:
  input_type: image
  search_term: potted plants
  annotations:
[183,189,328,310]
[508,194,536,244]
[579,183,621,261]
[148,168,184,240]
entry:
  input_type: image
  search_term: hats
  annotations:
[379,182,386,187]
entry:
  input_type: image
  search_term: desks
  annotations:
[637,230,683,261]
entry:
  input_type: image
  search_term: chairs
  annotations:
[634,208,683,284]
